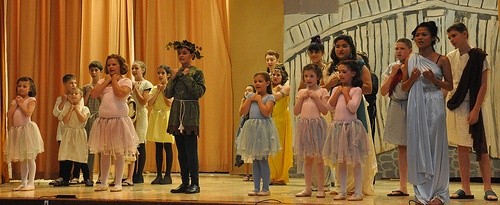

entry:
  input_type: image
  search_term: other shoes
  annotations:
[185,183,201,194]
[11,184,26,191]
[84,178,94,187]
[110,186,123,192]
[150,178,162,185]
[69,178,80,184]
[20,184,35,191]
[95,176,102,184]
[333,193,347,201]
[316,190,326,198]
[346,194,364,200]
[49,178,63,185]
[54,180,70,187]
[159,178,173,185]
[247,190,257,196]
[170,183,188,193]
[109,180,116,186]
[257,191,270,196]
[81,179,95,184]
[295,189,312,197]
[94,186,109,191]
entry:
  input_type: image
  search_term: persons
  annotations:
[235,72,282,195]
[48,60,105,187]
[163,39,206,194]
[234,50,292,185]
[446,22,498,201]
[379,38,414,196]
[88,55,132,192]
[109,59,177,186]
[293,35,379,201]
[5,77,44,192]
[401,21,454,205]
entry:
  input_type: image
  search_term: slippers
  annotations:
[483,189,498,202]
[386,190,410,197]
[122,180,135,186]
[448,188,475,199]
